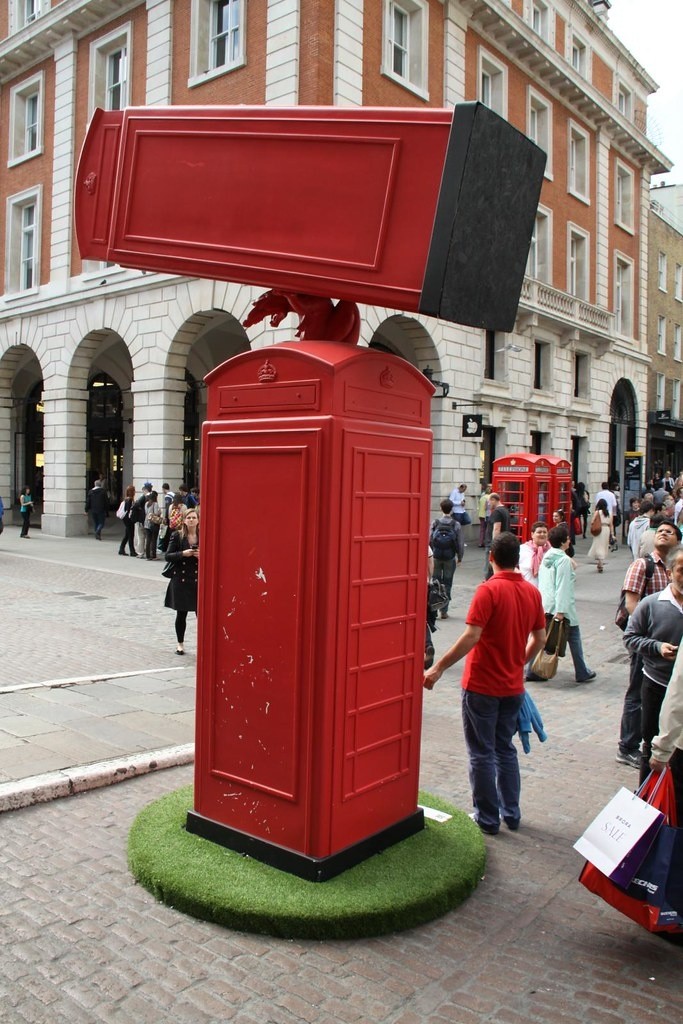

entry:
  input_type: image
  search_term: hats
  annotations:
[144,481,154,490]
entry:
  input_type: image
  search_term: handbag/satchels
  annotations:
[590,510,602,536]
[162,531,182,578]
[531,616,563,680]
[542,612,571,658]
[462,512,471,525]
[572,760,683,933]
[574,517,582,535]
[148,502,163,525]
[428,579,449,612]
[116,498,133,519]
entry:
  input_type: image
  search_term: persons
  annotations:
[20,487,34,538]
[429,499,463,619]
[616,520,682,768]
[625,472,683,560]
[0,496,5,534]
[119,483,200,561]
[588,482,622,573]
[539,481,591,538]
[424,545,434,669]
[515,511,597,682]
[649,637,683,945]
[84,473,109,539]
[424,532,546,835]
[165,509,199,654]
[478,488,511,582]
[622,548,683,786]
[449,484,471,546]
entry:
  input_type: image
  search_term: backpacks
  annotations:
[169,504,182,530]
[432,518,455,560]
[615,554,655,630]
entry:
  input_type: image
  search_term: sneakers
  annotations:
[617,750,644,769]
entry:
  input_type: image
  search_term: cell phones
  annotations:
[192,545,198,550]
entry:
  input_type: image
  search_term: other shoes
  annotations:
[425,646,435,669]
[119,547,159,561]
[176,646,184,655]
[478,544,485,548]
[597,565,603,572]
[469,812,498,835]
[441,612,448,619]
[94,531,101,541]
[20,533,30,539]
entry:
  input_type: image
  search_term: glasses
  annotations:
[655,528,678,537]
